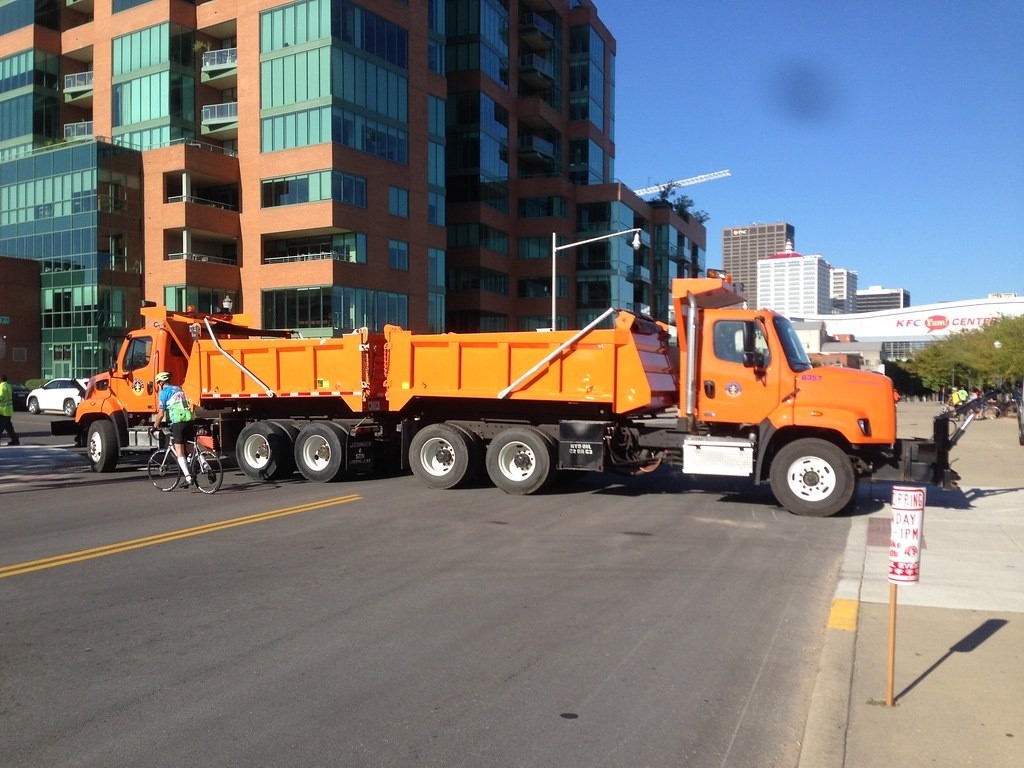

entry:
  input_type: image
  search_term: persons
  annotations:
[0,373,21,446]
[950,385,984,421]
[147,371,218,489]
[893,388,901,409]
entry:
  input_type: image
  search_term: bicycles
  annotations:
[943,399,1017,421]
[147,427,223,494]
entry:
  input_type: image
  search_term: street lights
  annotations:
[552,228,641,332]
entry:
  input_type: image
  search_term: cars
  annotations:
[11,383,30,412]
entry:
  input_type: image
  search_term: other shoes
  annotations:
[8,441,19,445]
[203,465,216,484]
[178,478,193,487]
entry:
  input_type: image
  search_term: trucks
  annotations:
[383,269,999,518]
[74,300,397,482]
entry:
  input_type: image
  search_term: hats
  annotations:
[952,388,957,391]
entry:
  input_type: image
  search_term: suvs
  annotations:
[26,378,91,417]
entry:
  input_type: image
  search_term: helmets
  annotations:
[155,372,171,382]
